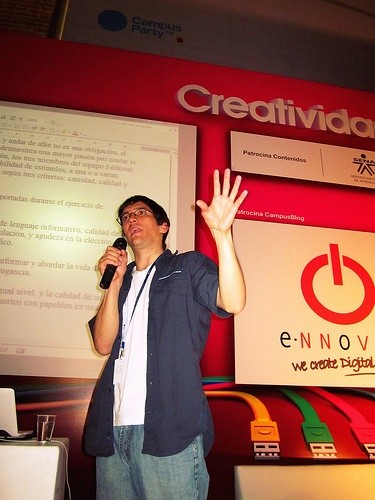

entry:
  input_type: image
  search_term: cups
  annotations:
[36,415,57,444]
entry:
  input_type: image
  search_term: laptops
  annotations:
[0,388,33,440]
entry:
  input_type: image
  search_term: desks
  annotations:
[0,437,69,500]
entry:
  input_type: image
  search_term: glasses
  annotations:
[116,208,154,226]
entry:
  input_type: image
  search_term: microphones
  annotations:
[100,238,127,289]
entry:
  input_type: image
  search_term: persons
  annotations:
[81,167,248,499]
